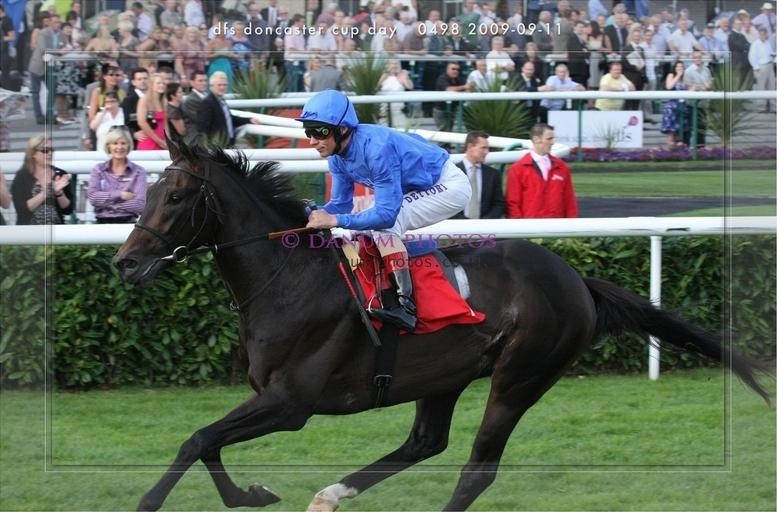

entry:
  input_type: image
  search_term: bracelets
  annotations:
[56,191,65,198]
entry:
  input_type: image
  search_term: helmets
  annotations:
[294,89,360,129]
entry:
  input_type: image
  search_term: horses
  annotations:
[110,130,777,512]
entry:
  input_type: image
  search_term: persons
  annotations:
[0,1,776,151]
[0,168,11,225]
[12,135,76,225]
[89,126,146,224]
[503,122,579,218]
[451,132,505,219]
[305,90,472,332]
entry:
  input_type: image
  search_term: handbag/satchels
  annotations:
[129,111,158,131]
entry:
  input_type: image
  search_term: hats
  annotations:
[760,3,775,9]
[737,9,750,17]
[705,21,715,29]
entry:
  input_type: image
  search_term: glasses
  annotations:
[105,73,121,78]
[36,147,55,154]
[304,126,332,141]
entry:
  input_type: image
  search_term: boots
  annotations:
[366,250,418,332]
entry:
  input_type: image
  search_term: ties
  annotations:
[540,157,548,180]
[465,165,480,219]
